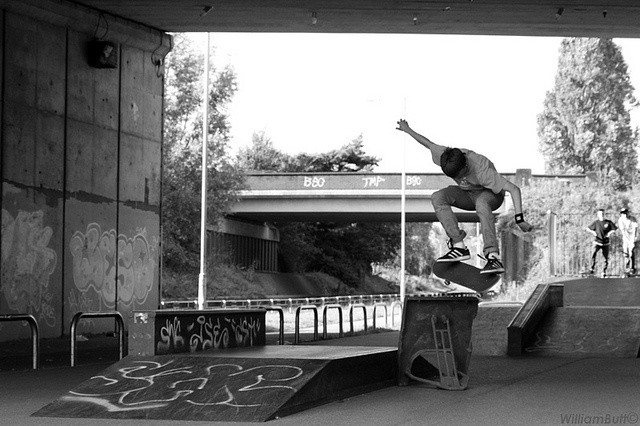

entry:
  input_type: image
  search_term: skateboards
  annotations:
[433,259,501,298]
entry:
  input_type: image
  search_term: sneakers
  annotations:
[436,247,470,262]
[477,254,505,274]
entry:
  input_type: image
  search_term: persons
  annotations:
[395,118,534,275]
[618,208,639,275]
[585,208,618,278]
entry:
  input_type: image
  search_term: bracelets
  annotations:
[515,213,524,224]
